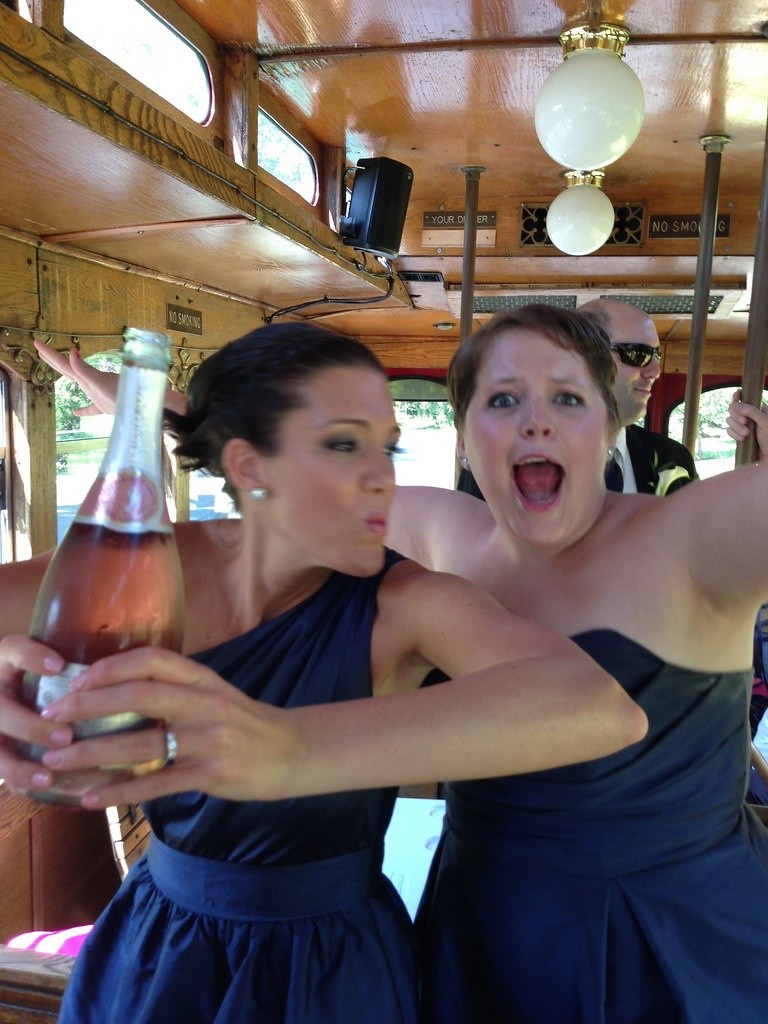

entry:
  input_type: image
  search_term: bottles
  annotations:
[14,326,184,807]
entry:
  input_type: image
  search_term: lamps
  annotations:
[534,22,645,171]
[545,171,616,256]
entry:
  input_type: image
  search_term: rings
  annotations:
[161,726,176,766]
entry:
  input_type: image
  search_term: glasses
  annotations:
[609,342,662,368]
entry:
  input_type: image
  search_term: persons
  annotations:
[0,323,647,1023]
[457,299,699,498]
[35,301,768,1024]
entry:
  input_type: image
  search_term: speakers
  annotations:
[342,156,414,260]
